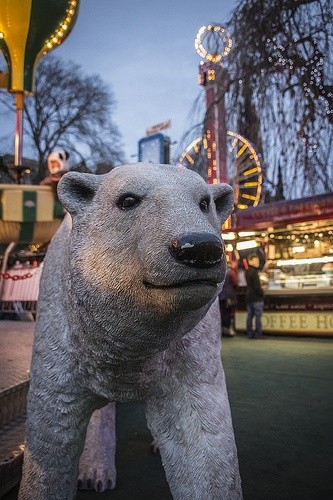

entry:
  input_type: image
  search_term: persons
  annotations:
[219,266,240,337]
[245,254,264,340]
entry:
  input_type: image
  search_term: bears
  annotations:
[17,163,244,500]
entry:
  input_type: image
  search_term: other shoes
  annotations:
[223,332,235,337]
[254,335,266,339]
[249,335,255,338]
